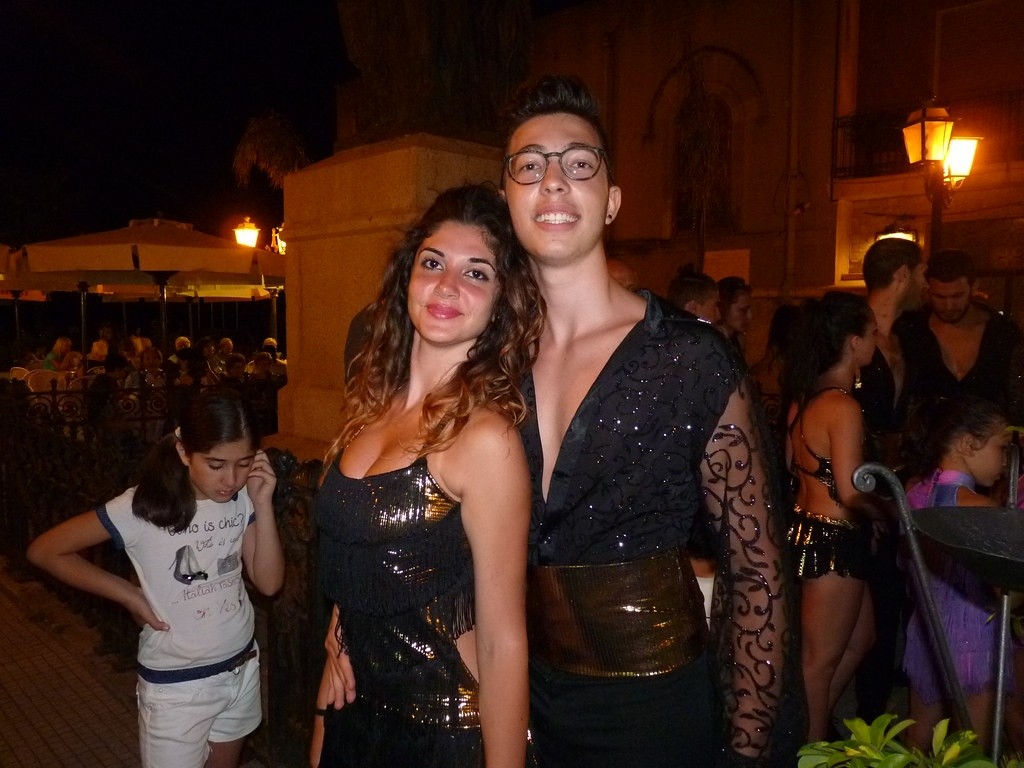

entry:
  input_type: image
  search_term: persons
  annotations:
[322,73,788,768]
[25,388,284,768]
[44,328,287,387]
[716,276,752,362]
[756,238,1023,756]
[309,183,547,768]
[671,268,721,325]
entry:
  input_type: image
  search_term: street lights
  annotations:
[894,94,988,267]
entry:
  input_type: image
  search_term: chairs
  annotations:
[8,353,252,456]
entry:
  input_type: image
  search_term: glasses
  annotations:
[499,143,610,186]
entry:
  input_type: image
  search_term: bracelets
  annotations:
[314,707,327,716]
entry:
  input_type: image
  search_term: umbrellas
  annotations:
[0,219,285,301]
